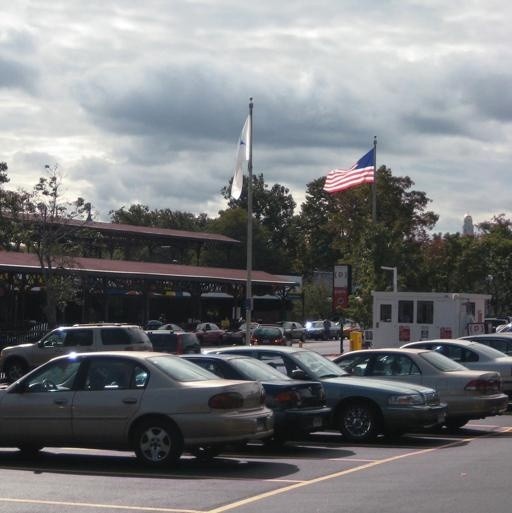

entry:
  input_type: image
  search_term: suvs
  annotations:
[147,322,202,355]
[0,324,154,383]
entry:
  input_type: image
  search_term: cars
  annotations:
[247,324,289,345]
[179,354,332,446]
[305,321,340,340]
[335,348,508,429]
[283,321,307,342]
[206,346,448,442]
[457,335,512,357]
[337,320,361,340]
[0,351,276,468]
[232,321,264,345]
[401,339,512,396]
[188,324,233,344]
